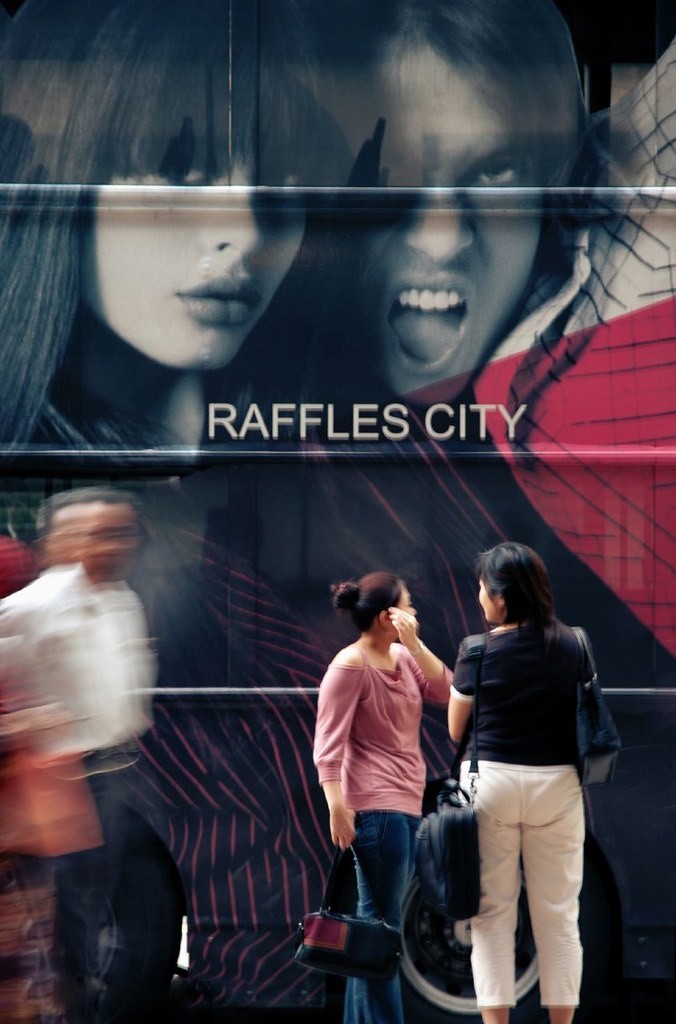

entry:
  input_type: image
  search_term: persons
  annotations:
[312,571,454,1024]
[0,483,158,1024]
[447,542,596,1024]
[0,0,591,672]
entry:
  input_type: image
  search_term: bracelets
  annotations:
[411,642,425,657]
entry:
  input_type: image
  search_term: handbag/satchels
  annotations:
[0,753,106,858]
[414,806,478,918]
[572,627,623,786]
[294,839,404,983]
